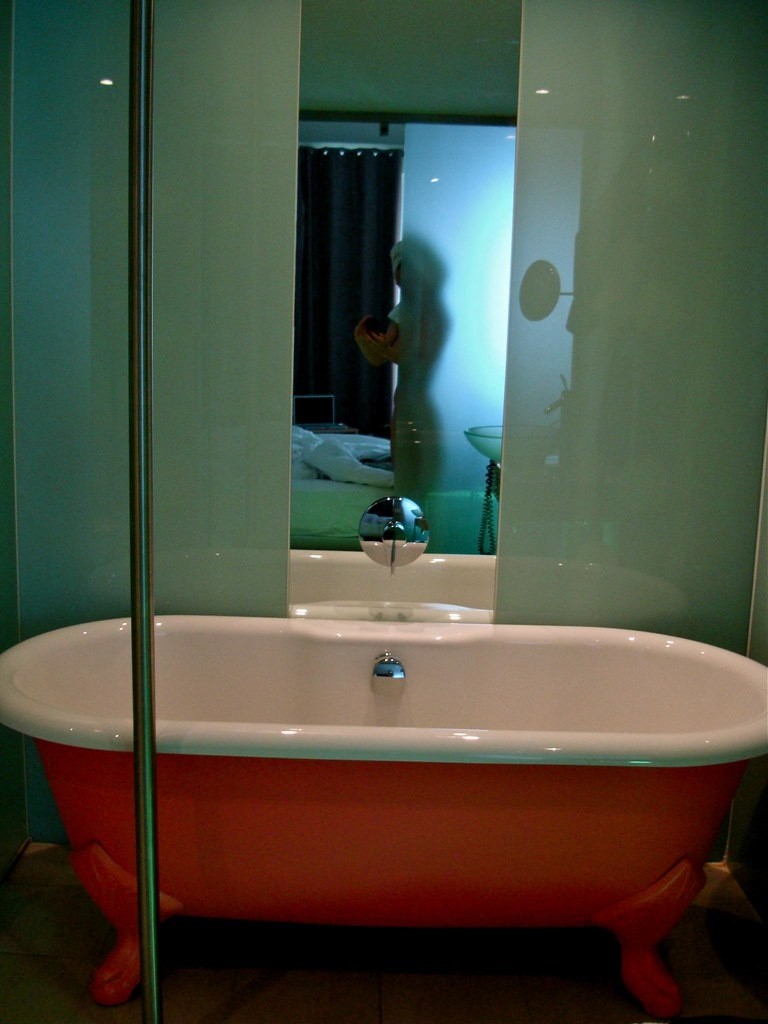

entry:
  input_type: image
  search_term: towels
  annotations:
[391,235,424,273]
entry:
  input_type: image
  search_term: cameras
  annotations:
[365,313,390,338]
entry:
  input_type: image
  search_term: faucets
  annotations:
[543,374,570,415]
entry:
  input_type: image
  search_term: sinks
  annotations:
[463,424,544,464]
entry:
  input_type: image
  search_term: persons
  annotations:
[355,240,451,504]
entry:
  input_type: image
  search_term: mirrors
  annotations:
[287,1,522,626]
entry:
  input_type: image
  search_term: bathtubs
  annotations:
[82,547,686,631]
[3,612,765,928]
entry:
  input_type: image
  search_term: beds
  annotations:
[290,424,393,552]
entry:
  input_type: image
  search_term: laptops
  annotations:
[294,395,347,430]
